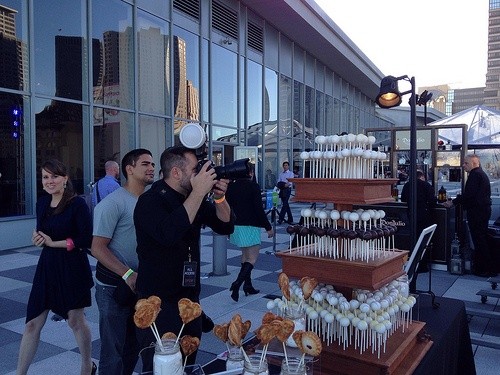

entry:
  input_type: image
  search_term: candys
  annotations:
[267,273,416,359]
[286,209,397,263]
[300,133,386,180]
[134,296,202,372]
[214,312,322,372]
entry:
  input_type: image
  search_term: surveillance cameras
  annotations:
[228,40,232,45]
[346,95,349,99]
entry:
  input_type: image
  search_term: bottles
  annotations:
[243,354,270,375]
[226,349,244,370]
[281,358,307,375]
[152,338,182,375]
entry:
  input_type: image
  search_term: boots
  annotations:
[241,263,260,296]
[230,262,254,302]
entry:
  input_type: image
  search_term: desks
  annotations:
[193,294,478,375]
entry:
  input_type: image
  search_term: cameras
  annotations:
[197,158,251,180]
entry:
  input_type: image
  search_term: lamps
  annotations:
[376,74,412,108]
[343,96,348,99]
[223,37,232,45]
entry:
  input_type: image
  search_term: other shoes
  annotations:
[91,361,98,375]
[51,312,86,323]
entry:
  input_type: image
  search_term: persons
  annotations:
[444,154,491,277]
[277,161,295,225]
[92,161,120,206]
[16,158,96,375]
[400,170,436,273]
[227,161,274,302]
[133,146,235,375]
[383,172,388,178]
[90,148,155,375]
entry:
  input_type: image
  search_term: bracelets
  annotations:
[66,238,74,251]
[215,195,225,203]
[122,269,133,279]
